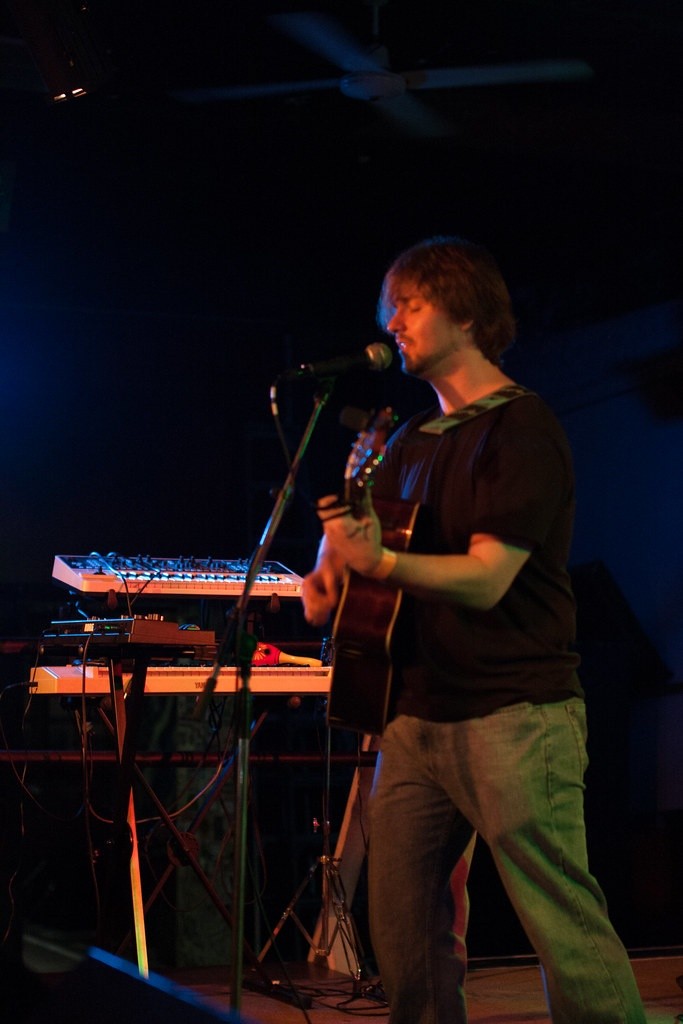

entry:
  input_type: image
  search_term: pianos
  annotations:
[26,659,331,698]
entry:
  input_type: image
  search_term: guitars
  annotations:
[323,407,419,738]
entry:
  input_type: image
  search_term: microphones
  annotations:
[299,343,392,376]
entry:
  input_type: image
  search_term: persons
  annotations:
[303,237,649,1024]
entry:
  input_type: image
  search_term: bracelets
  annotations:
[373,548,397,581]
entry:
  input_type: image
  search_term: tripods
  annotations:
[242,725,364,1001]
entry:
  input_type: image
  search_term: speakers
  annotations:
[0,928,248,1024]
